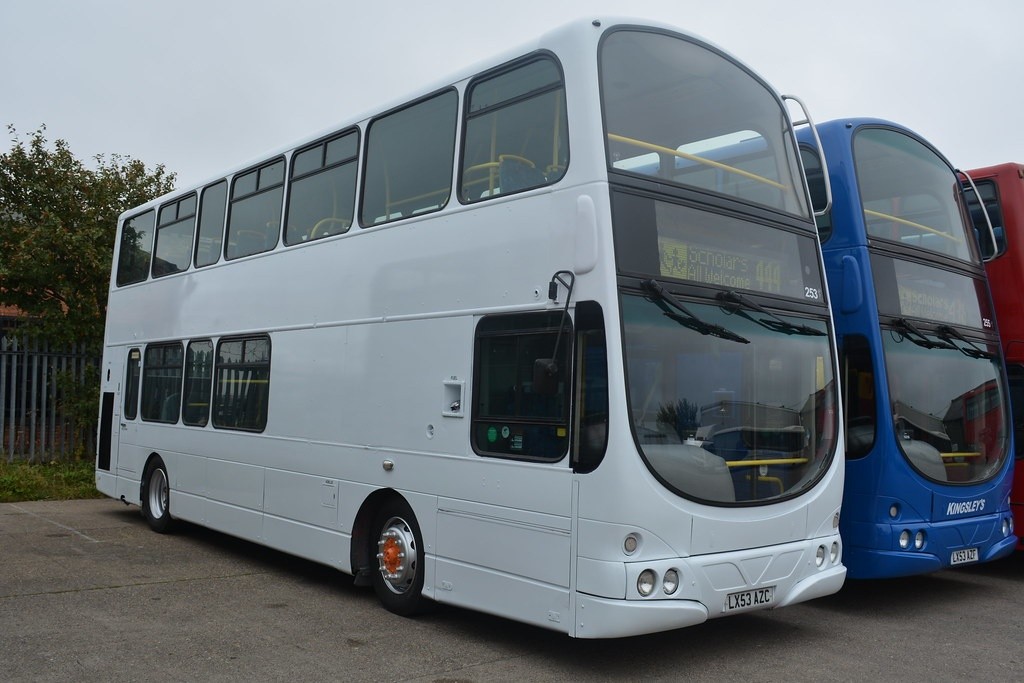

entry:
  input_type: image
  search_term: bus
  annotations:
[90,14,849,646]
[864,160,1023,553]
[479,115,1019,584]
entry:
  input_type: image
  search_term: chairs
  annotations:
[497,160,567,195]
[163,391,256,429]
